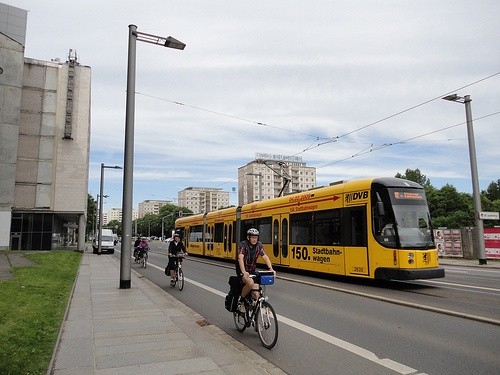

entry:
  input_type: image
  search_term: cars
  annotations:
[132,230,174,243]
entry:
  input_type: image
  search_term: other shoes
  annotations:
[237,301,246,313]
[170,279,174,283]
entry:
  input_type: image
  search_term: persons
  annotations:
[138,237,150,263]
[381,216,399,236]
[235,227,277,332]
[134,237,141,264]
[437,243,445,255]
[167,233,189,283]
[435,231,444,239]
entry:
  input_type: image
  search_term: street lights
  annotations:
[441,94,487,266]
[95,194,109,232]
[98,163,122,254]
[120,24,186,289]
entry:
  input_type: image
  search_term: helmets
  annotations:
[174,234,180,237]
[247,228,260,236]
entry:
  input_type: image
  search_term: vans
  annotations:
[114,234,123,246]
[92,229,114,254]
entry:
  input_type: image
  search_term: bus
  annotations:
[175,178,444,285]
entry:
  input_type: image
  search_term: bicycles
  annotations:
[168,253,188,291]
[229,271,278,349]
[134,246,150,269]
